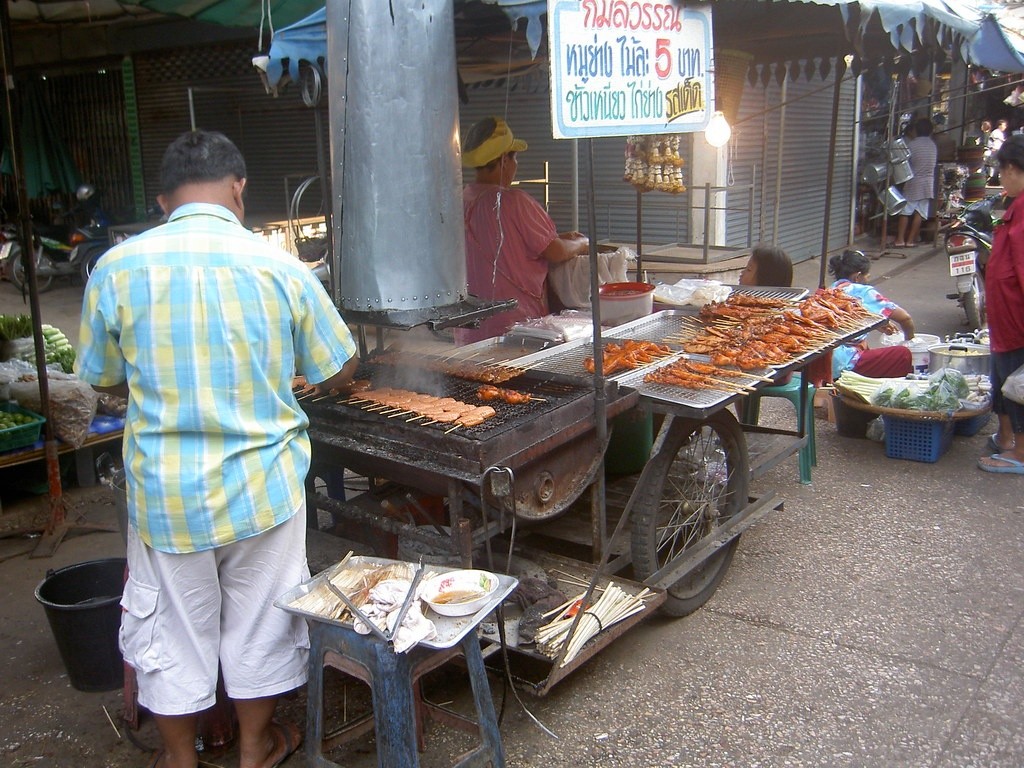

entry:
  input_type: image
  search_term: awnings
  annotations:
[960,13,1024,74]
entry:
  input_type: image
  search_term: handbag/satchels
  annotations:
[1001,364,1024,406]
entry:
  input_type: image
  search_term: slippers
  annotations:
[269,719,302,768]
[988,434,1014,453]
[977,455,1024,474]
[906,242,920,248]
[886,242,905,249]
[148,751,165,768]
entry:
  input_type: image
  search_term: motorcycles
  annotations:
[0,182,119,289]
[938,184,1006,330]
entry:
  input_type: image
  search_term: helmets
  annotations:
[76,184,100,202]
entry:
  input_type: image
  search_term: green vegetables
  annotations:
[873,368,969,414]
[0,312,34,340]
[43,347,77,375]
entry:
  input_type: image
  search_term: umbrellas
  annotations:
[251,0,982,235]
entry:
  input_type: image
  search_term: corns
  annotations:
[41,324,73,352]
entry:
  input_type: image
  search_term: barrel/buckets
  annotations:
[877,185,907,217]
[892,160,914,184]
[862,162,888,187]
[33,556,128,692]
[880,135,912,164]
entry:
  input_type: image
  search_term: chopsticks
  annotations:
[534,580,650,669]
[288,549,439,623]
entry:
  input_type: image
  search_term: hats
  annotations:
[462,116,528,168]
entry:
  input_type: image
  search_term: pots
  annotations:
[96,452,127,550]
[927,333,991,376]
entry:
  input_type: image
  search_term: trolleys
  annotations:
[277,265,887,691]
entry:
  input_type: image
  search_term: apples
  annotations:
[0,410,38,441]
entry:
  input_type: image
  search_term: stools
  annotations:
[303,620,506,768]
[736,372,816,484]
[815,388,837,423]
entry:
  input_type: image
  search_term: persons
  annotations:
[72,131,358,768]
[976,119,1024,150]
[683,245,793,445]
[976,134,1024,474]
[888,118,937,247]
[824,249,915,379]
[454,117,589,350]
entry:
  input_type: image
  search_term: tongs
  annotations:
[322,553,425,648]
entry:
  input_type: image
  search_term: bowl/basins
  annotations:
[957,145,987,207]
[598,282,656,327]
[420,569,500,617]
[907,333,941,374]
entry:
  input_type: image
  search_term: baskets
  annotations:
[0,404,47,452]
[955,413,991,437]
[880,416,953,462]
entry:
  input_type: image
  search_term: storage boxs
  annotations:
[884,410,990,462]
